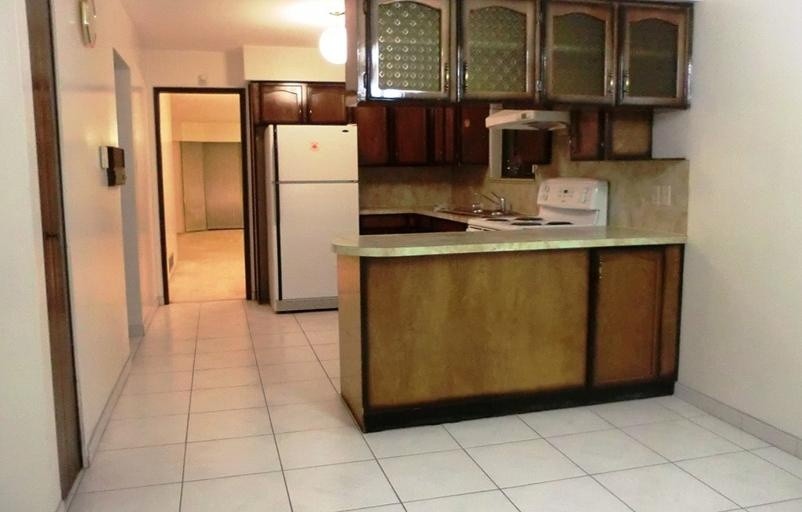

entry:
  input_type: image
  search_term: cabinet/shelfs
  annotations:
[569,105,687,160]
[247,79,354,125]
[359,214,467,235]
[352,100,553,165]
[356,0,695,108]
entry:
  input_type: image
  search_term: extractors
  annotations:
[484,106,572,132]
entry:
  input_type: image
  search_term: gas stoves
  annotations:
[467,214,577,231]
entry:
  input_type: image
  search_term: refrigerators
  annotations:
[263,123,360,314]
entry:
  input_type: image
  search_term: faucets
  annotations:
[480,192,505,211]
